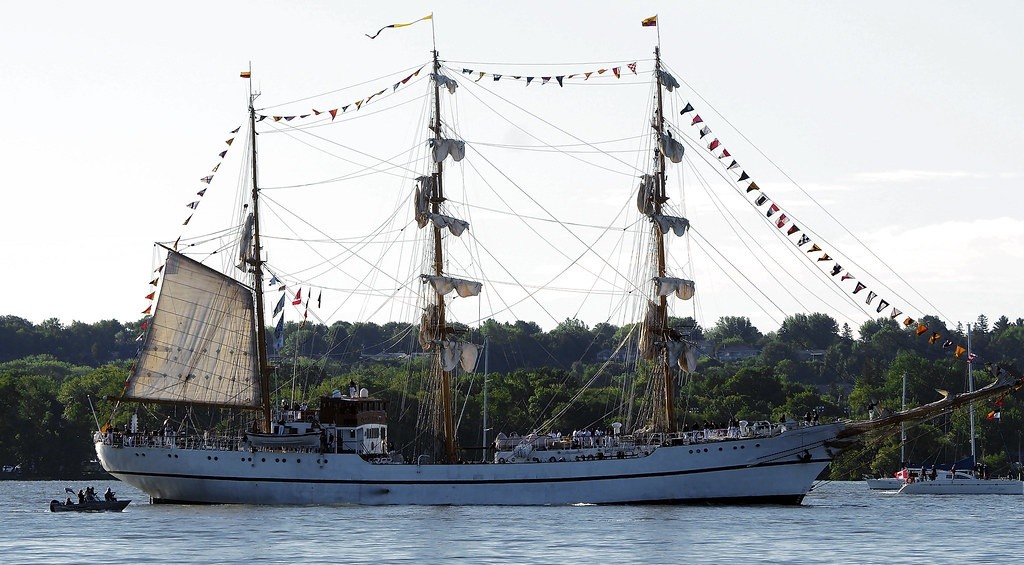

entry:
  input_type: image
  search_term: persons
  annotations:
[105,425,172,446]
[328,432,334,452]
[683,418,741,439]
[349,378,357,399]
[550,427,610,449]
[779,409,819,427]
[867,401,879,421]
[78,486,116,505]
[908,464,987,484]
[65,498,74,505]
[389,442,395,461]
[281,400,307,420]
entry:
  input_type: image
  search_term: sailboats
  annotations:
[85,44,1023,507]
[865,318,1024,495]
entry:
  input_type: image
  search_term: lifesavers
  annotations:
[549,457,556,462]
[510,457,516,463]
[360,417,364,422]
[906,477,913,483]
[307,415,314,423]
[499,458,505,464]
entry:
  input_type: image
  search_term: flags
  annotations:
[680,102,976,364]
[894,469,907,480]
[641,16,656,27]
[269,276,322,351]
[240,72,250,78]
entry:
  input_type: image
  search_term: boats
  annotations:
[50,487,132,514]
